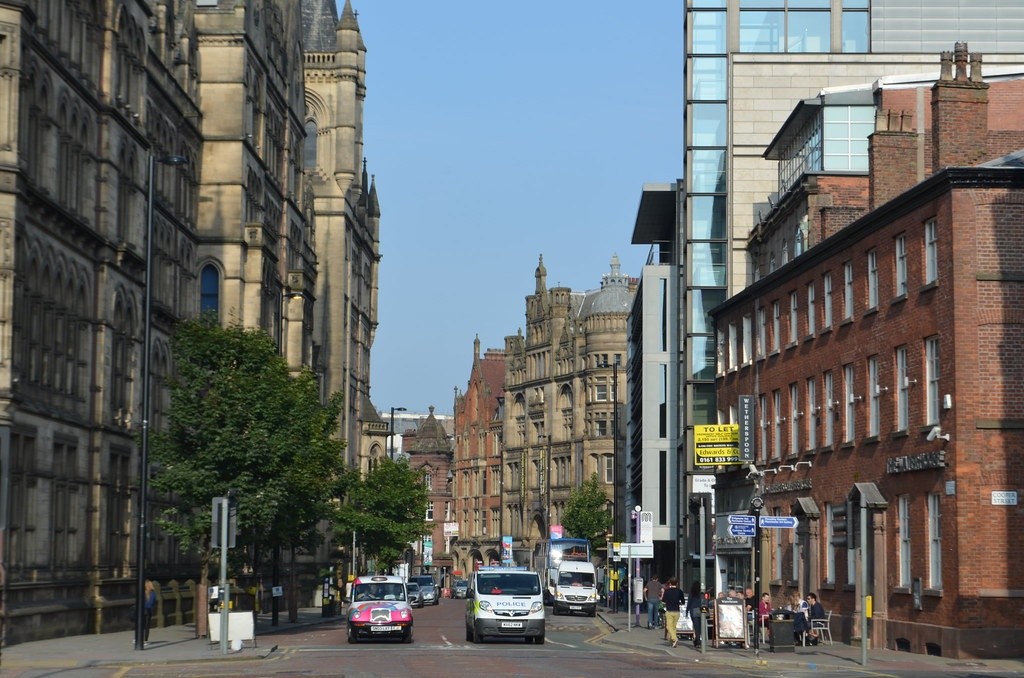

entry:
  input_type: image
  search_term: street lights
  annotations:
[136,149,186,641]
[390,407,407,460]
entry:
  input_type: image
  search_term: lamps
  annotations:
[745,461,812,479]
[760,377,917,427]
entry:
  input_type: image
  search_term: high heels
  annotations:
[796,642,801,646]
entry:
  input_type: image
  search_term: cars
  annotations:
[398,583,424,609]
[464,566,547,645]
[349,575,413,640]
[553,560,597,617]
[408,575,440,606]
[450,580,472,598]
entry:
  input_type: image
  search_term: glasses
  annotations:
[807,598,810,600]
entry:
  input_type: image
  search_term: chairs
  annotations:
[707,608,833,647]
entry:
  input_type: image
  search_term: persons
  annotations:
[686,582,825,648]
[643,575,686,648]
[131,580,155,645]
[620,573,630,613]
[357,586,376,601]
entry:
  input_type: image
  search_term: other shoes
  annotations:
[672,638,678,648]
[695,644,700,648]
[655,626,659,629]
[649,623,652,630]
[143,641,151,646]
[133,640,135,644]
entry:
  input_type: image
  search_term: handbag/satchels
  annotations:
[805,633,819,646]
[693,607,701,617]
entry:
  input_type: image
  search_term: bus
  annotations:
[532,538,589,603]
